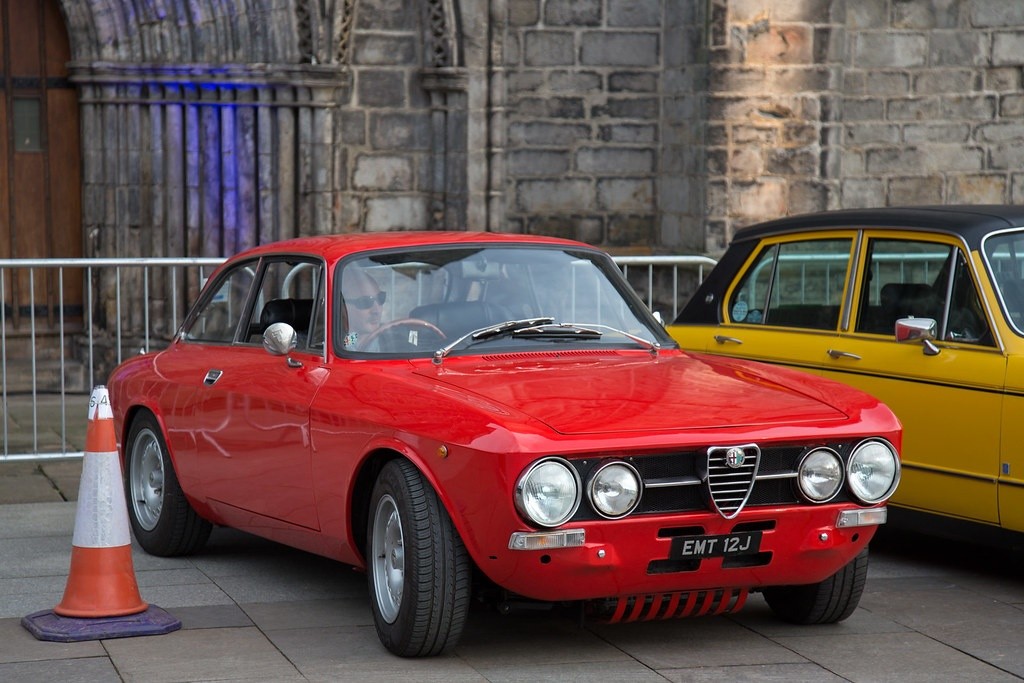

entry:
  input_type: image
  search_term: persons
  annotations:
[310,268,420,354]
[930,262,1006,347]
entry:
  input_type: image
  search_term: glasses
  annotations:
[342,292,387,311]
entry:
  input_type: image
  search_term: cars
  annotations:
[106,231,904,658]
[666,204,1023,535]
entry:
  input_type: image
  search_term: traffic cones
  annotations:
[20,385,181,643]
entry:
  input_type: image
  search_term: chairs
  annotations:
[409,301,486,337]
[260,299,314,333]
[880,283,937,317]
